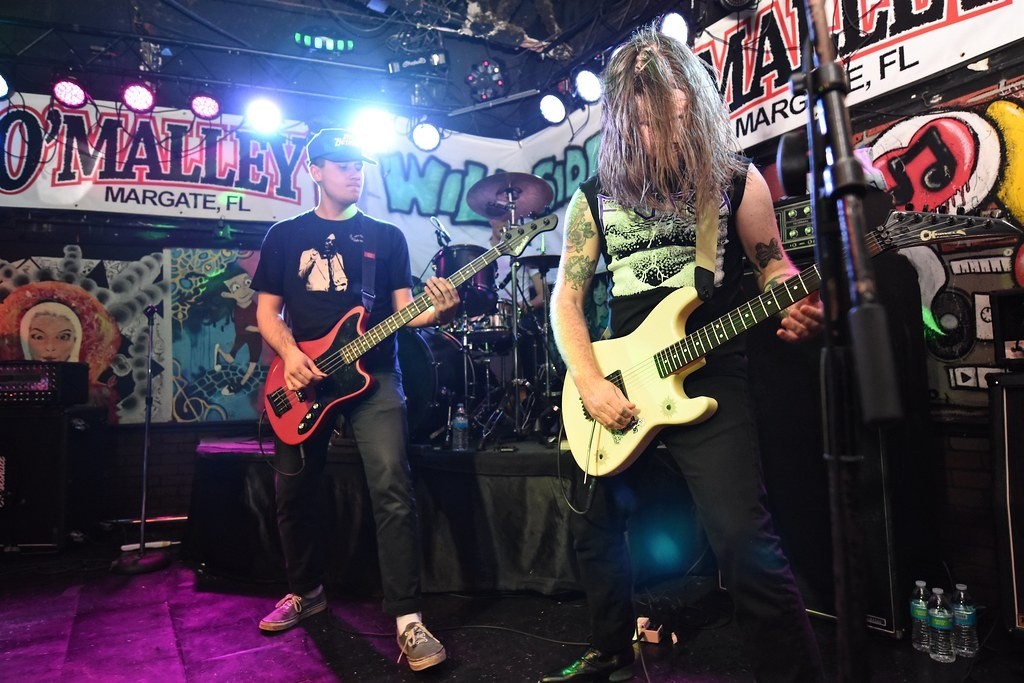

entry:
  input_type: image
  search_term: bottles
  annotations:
[928,588,955,662]
[910,580,931,651]
[453,403,469,452]
[952,584,979,657]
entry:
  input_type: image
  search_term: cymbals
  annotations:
[466,172,555,222]
[515,252,561,268]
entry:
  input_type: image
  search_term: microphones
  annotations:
[498,264,521,290]
[436,220,451,242]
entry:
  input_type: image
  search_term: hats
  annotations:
[307,128,378,165]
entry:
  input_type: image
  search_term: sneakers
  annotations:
[259,588,328,630]
[397,620,447,671]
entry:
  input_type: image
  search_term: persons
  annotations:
[538,21,827,683]
[249,129,462,670]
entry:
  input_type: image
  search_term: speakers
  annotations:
[742,251,937,640]
[0,402,109,553]
[985,370,1024,637]
[193,440,291,602]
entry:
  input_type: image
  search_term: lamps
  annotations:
[409,117,443,151]
[51,69,92,109]
[658,5,709,49]
[0,61,17,102]
[573,64,603,104]
[538,87,577,126]
[190,82,226,120]
[120,77,160,114]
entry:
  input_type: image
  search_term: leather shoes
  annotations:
[537,645,635,683]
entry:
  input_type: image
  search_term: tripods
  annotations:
[428,192,565,455]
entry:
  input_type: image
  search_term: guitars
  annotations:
[561,201,1024,478]
[264,205,558,447]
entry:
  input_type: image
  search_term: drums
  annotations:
[449,297,512,342]
[433,244,497,317]
